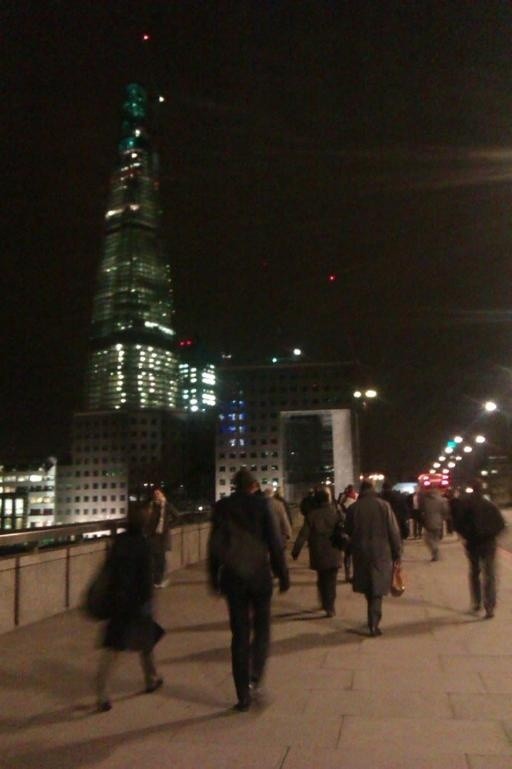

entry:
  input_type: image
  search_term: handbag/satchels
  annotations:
[392,561,405,597]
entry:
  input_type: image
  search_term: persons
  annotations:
[206,469,291,714]
[456,477,505,618]
[148,488,180,589]
[94,502,166,712]
[265,477,469,636]
